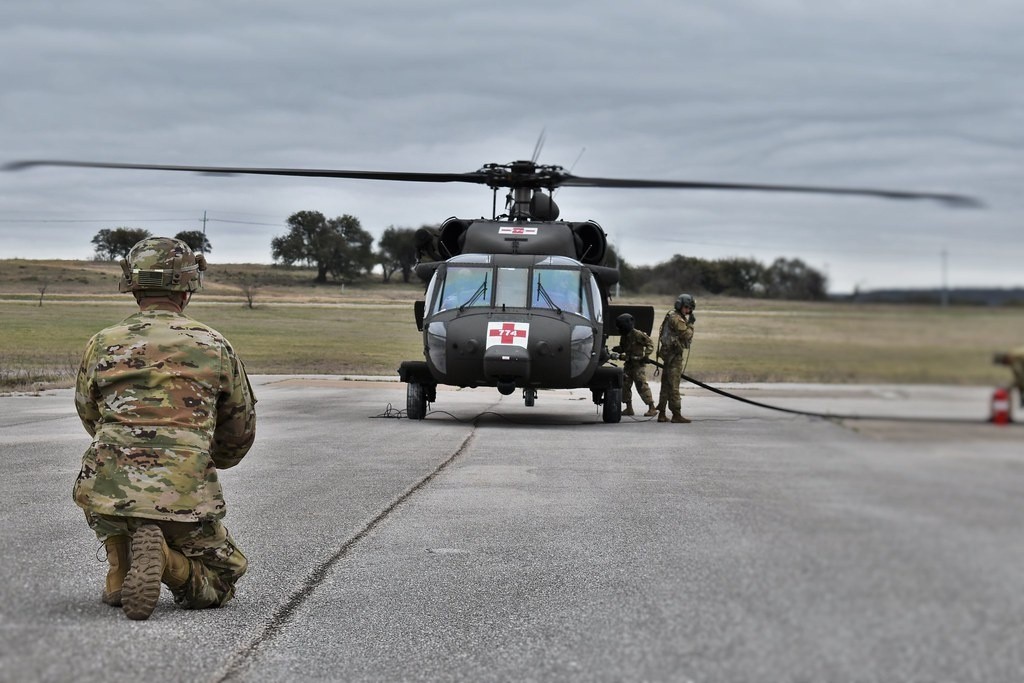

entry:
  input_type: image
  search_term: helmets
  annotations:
[616,313,634,334]
[118,236,202,292]
[674,293,695,311]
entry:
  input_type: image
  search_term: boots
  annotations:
[120,523,191,620]
[96,533,134,606]
[645,402,658,415]
[621,402,634,415]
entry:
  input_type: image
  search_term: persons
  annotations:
[543,277,580,313]
[613,312,659,418]
[991,347,1024,411]
[658,293,697,423]
[74,236,258,621]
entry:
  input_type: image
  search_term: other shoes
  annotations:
[671,413,692,423]
[658,415,670,421]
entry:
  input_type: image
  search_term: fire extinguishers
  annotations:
[991,390,1010,424]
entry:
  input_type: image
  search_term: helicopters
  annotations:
[0,127,991,422]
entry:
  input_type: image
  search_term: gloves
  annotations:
[612,347,617,352]
[688,313,696,323]
[642,354,649,361]
